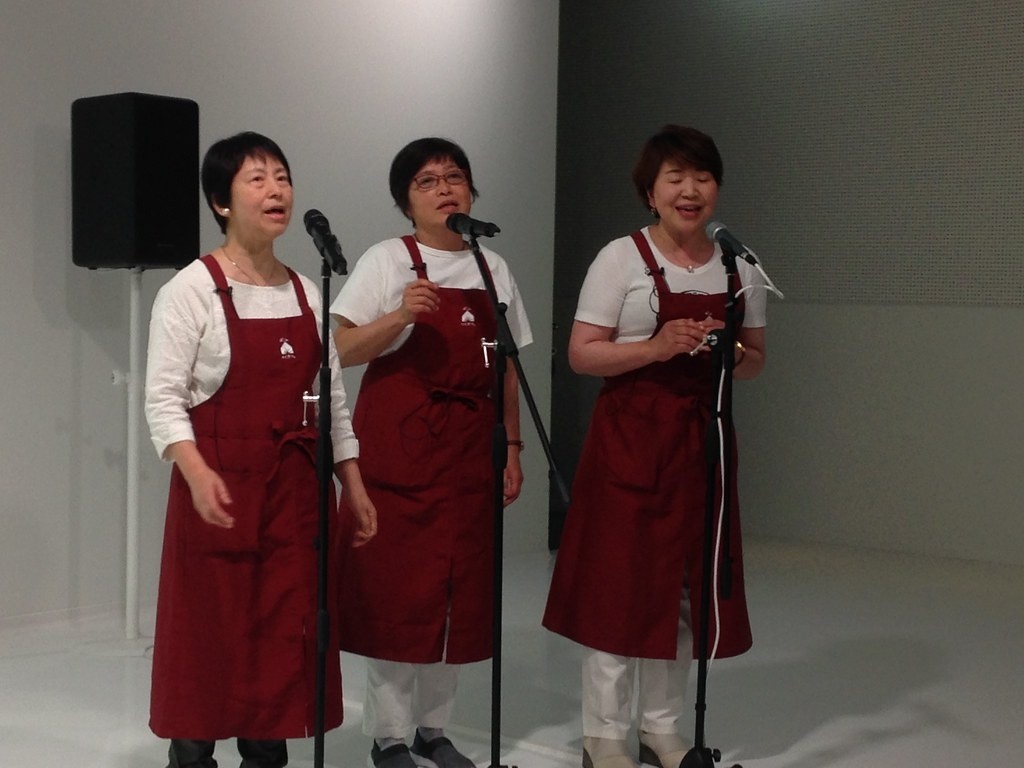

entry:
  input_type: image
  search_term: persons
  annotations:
[143,131,379,768]
[540,122,769,768]
[327,137,535,768]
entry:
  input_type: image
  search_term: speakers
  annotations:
[72,93,201,270]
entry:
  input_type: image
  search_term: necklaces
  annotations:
[413,233,466,251]
[221,245,277,286]
[652,237,703,273]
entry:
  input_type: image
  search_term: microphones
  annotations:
[445,213,500,237]
[303,208,348,275]
[705,220,758,266]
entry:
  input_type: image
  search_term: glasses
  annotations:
[412,170,466,192]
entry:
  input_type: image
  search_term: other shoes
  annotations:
[410,727,477,768]
[370,738,418,768]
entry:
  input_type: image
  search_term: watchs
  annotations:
[733,340,746,367]
[507,440,524,450]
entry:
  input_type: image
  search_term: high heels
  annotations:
[638,739,715,768]
[581,746,636,768]
